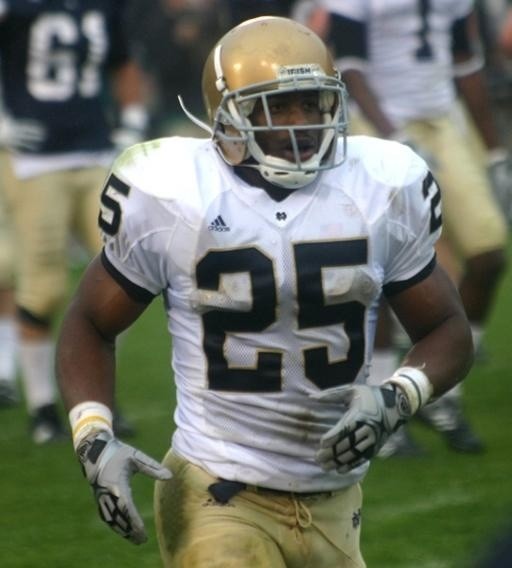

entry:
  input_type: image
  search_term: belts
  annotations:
[206,476,330,506]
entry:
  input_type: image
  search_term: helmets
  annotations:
[201,16,347,190]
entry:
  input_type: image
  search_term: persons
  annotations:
[52,13,472,567]
[1,1,511,460]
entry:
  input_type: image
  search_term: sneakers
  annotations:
[0,379,22,408]
[414,397,484,454]
[30,406,68,444]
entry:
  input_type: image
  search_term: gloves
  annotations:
[309,366,435,475]
[69,401,173,545]
